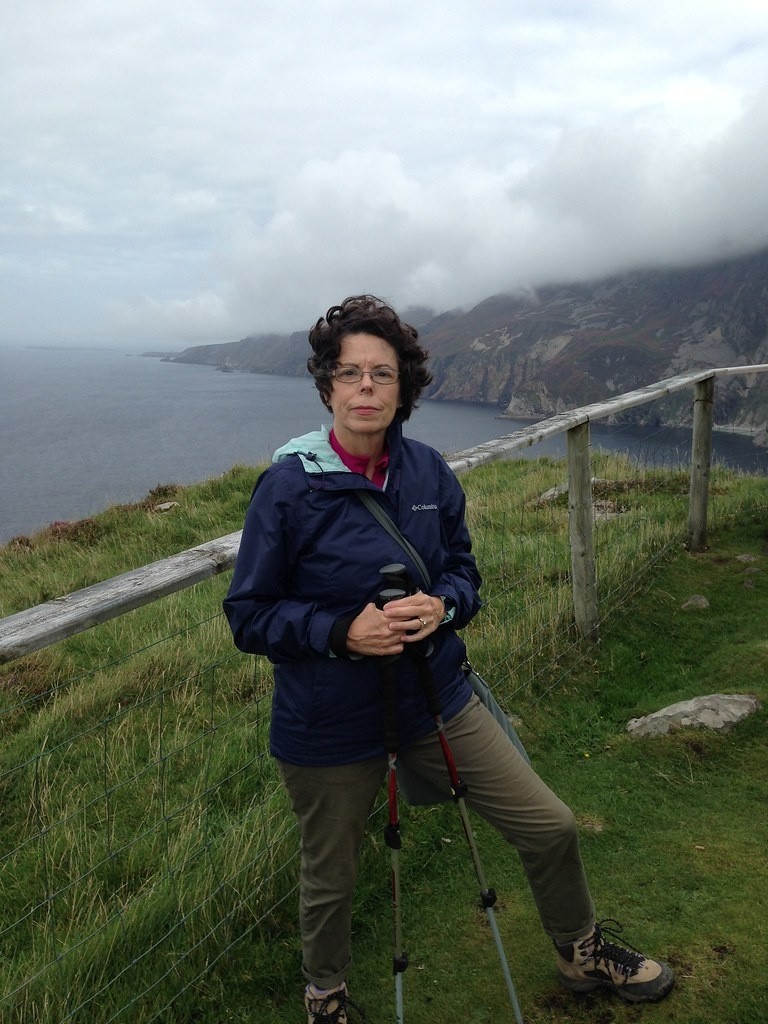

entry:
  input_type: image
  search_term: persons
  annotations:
[222,295,676,1024]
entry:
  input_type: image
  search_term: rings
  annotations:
[418,618,427,629]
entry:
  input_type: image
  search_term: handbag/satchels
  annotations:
[394,662,531,806]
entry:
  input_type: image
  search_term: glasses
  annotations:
[332,365,401,385]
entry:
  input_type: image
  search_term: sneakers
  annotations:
[553,919,672,1002]
[304,981,349,1024]
